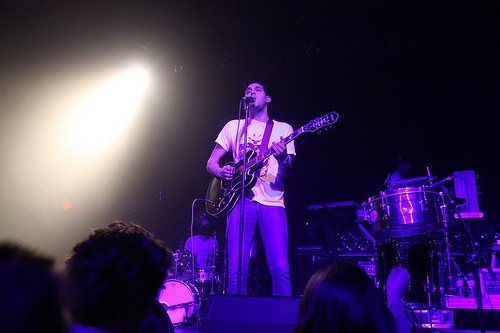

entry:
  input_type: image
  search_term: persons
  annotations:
[184,220,219,325]
[0,220,177,333]
[296,263,420,333]
[205,81,297,297]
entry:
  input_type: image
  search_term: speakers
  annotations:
[297,252,379,292]
[203,293,304,333]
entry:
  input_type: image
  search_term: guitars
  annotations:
[205,110,340,219]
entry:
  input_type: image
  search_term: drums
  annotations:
[158,268,219,326]
[363,175,438,239]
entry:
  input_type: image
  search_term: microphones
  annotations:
[242,96,255,103]
[429,175,456,189]
